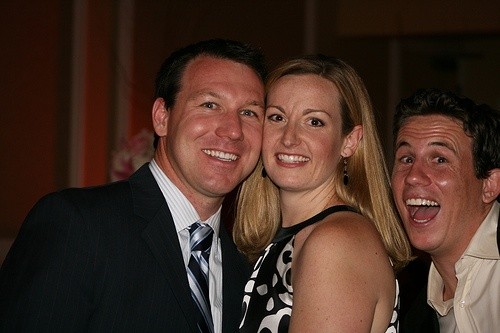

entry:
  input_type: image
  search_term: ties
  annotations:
[186,222,214,333]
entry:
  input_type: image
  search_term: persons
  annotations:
[236,54,417,333]
[390,91,500,333]
[0,40,266,333]
